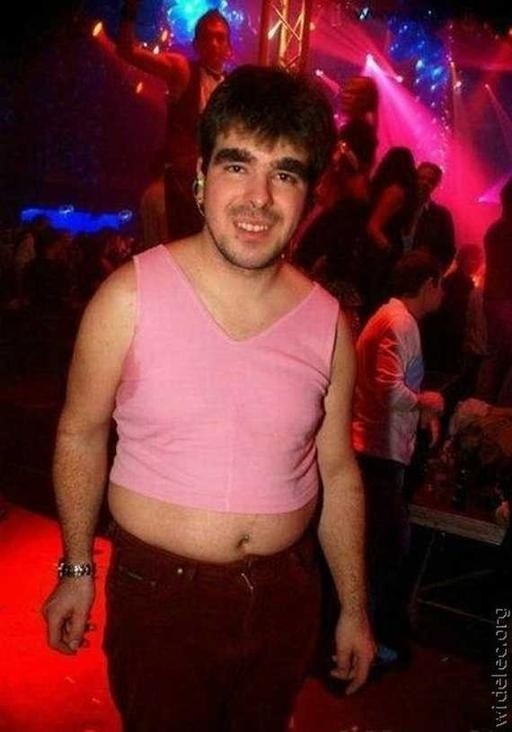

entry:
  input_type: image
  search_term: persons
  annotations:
[0,0,512,732]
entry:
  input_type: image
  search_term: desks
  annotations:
[392,470,510,705]
